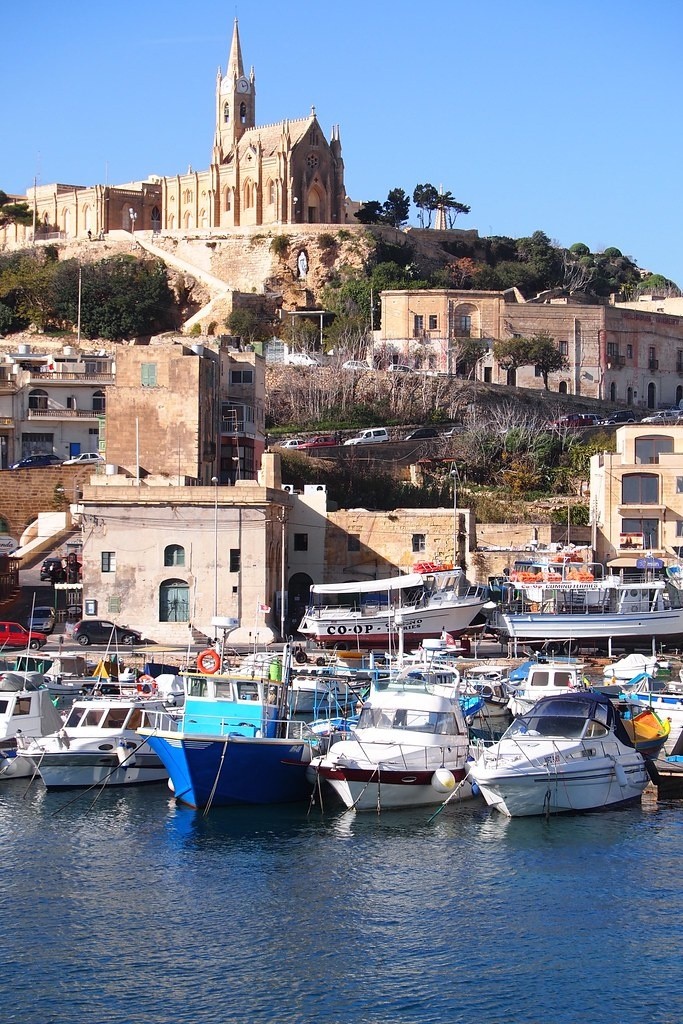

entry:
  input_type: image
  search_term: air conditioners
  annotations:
[304,485,326,494]
[281,485,294,494]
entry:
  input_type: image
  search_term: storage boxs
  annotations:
[666,755,683,763]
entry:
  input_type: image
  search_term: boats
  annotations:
[0,483,683,821]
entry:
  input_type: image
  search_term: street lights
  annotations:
[211,476,222,641]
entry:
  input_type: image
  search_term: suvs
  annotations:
[0,622,48,650]
[40,556,67,581]
[297,434,336,449]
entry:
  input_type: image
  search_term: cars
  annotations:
[71,619,141,645]
[640,404,683,423]
[42,454,66,465]
[399,427,439,441]
[62,453,104,465]
[387,364,419,375]
[438,427,466,439]
[26,606,56,633]
[7,453,67,469]
[342,361,377,372]
[277,439,304,450]
[286,352,321,367]
[545,412,593,430]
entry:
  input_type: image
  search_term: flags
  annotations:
[445,632,455,646]
[258,601,271,614]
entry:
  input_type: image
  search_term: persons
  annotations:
[267,687,277,704]
[49,560,82,585]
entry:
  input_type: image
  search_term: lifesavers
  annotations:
[295,652,307,663]
[316,657,325,667]
[137,675,157,699]
[197,650,220,674]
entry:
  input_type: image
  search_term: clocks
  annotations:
[238,80,248,92]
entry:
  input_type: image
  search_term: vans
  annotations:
[577,414,604,426]
[343,427,389,447]
[597,409,636,425]
